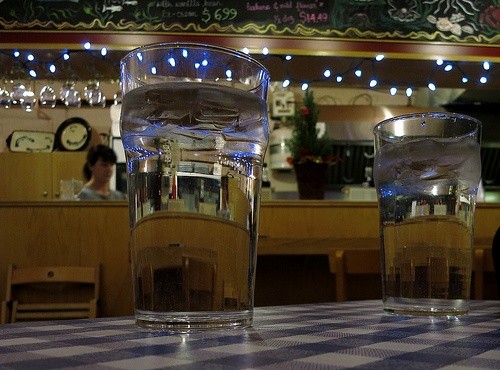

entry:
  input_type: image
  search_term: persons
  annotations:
[78,143,127,202]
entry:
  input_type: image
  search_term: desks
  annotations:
[0,299,500,370]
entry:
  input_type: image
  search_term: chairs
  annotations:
[327,247,483,301]
[1,262,100,325]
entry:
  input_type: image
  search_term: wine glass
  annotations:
[0,80,125,111]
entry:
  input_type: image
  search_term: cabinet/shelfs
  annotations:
[0,152,109,201]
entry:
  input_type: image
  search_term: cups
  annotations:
[119,42,270,335]
[372,112,483,320]
